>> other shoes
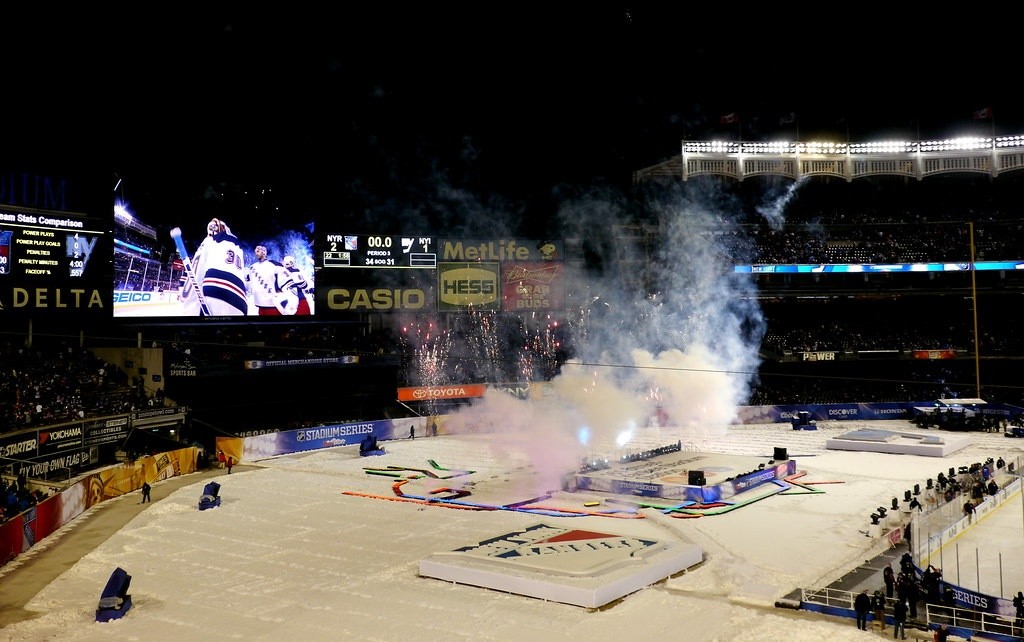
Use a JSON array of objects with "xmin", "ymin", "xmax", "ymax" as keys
[
  {"xmin": 862, "ymin": 628, "xmax": 867, "ymax": 631},
  {"xmin": 902, "ymin": 637, "xmax": 906, "ymax": 640}
]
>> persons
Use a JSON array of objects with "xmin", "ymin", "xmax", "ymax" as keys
[
  {"xmin": 227, "ymin": 457, "xmax": 232, "ymax": 474},
  {"xmin": 432, "ymin": 422, "xmax": 438, "ymax": 437},
  {"xmin": 904, "ymin": 524, "xmax": 911, "ymax": 552},
  {"xmin": 141, "ymin": 482, "xmax": 151, "ymax": 503},
  {"xmin": 913, "ymin": 405, "xmax": 1007, "ymax": 432},
  {"xmin": 715, "ymin": 209, "xmax": 1024, "ymax": 404},
  {"xmin": 113, "ymin": 207, "xmax": 312, "ymax": 315},
  {"xmin": 0, "ymin": 470, "xmax": 48, "ymax": 525},
  {"xmin": 408, "ymin": 425, "xmax": 414, "ymax": 439},
  {"xmin": 854, "ymin": 553, "xmax": 1024, "ymax": 642},
  {"xmin": 908, "ymin": 456, "xmax": 1004, "ymax": 526},
  {"xmin": 197, "ymin": 450, "xmax": 214, "ymax": 469},
  {"xmin": 219, "ymin": 450, "xmax": 226, "ymax": 469},
  {"xmin": 0, "ymin": 343, "xmax": 163, "ymax": 432}
]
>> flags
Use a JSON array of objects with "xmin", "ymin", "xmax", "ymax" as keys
[
  {"xmin": 685, "ymin": 115, "xmax": 706, "ymax": 128},
  {"xmin": 720, "ymin": 113, "xmax": 738, "ymax": 124},
  {"xmin": 974, "ymin": 109, "xmax": 992, "ymax": 118},
  {"xmin": 779, "ymin": 113, "xmax": 795, "ymax": 125}
]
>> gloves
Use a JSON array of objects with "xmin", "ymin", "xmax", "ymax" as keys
[{"xmin": 284, "ymin": 291, "xmax": 299, "ymax": 315}]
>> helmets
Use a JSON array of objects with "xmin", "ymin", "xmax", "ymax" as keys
[
  {"xmin": 283, "ymin": 256, "xmax": 295, "ymax": 268},
  {"xmin": 255, "ymin": 246, "xmax": 266, "ymax": 257},
  {"xmin": 206, "ymin": 218, "xmax": 226, "ymax": 236}
]
